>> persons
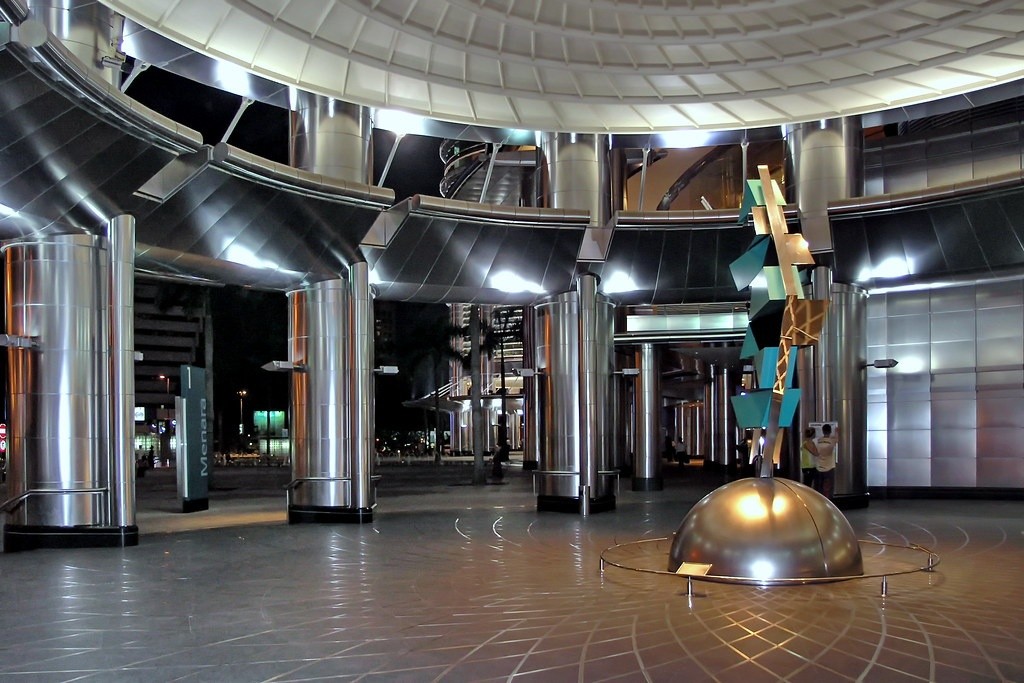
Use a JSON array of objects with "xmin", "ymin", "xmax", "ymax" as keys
[
  {"xmin": 137, "ymin": 445, "xmax": 143, "ymax": 461},
  {"xmin": 491, "ymin": 444, "xmax": 503, "ymax": 482},
  {"xmin": 816, "ymin": 424, "xmax": 839, "ymax": 501},
  {"xmin": 675, "ymin": 436, "xmax": 685, "ymax": 468},
  {"xmin": 148, "ymin": 445, "xmax": 154, "ymax": 469},
  {"xmin": 666, "ymin": 431, "xmax": 673, "ymax": 461},
  {"xmin": 137, "ymin": 456, "xmax": 147, "ymax": 478},
  {"xmin": 801, "ymin": 427, "xmax": 818, "ymax": 488}
]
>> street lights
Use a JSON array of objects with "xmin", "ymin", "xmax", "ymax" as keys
[
  {"xmin": 161, "ymin": 375, "xmax": 169, "ymax": 394},
  {"xmin": 237, "ymin": 391, "xmax": 247, "ymax": 424}
]
